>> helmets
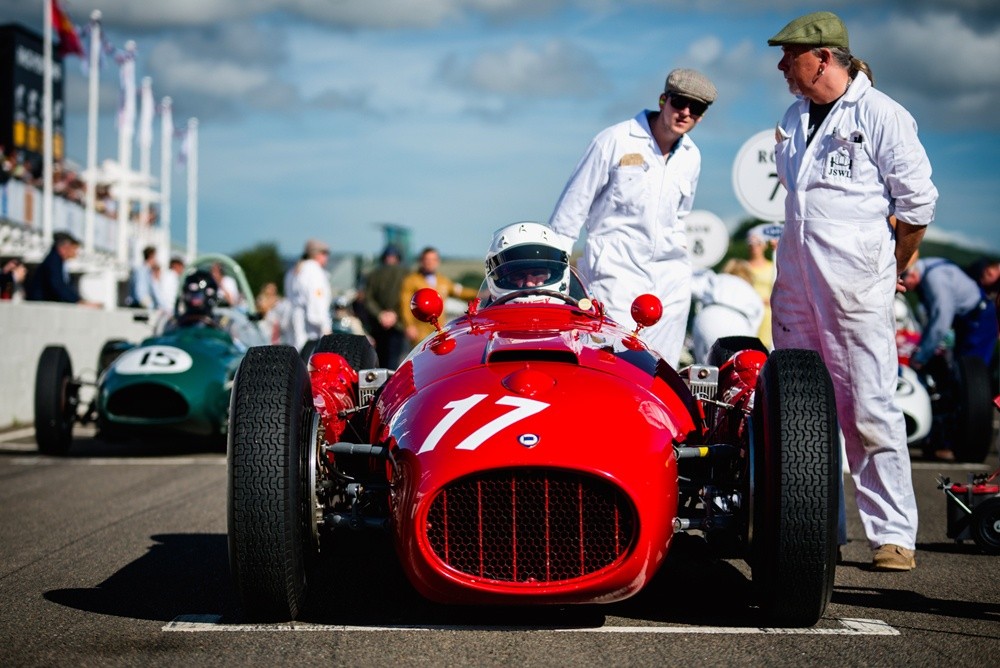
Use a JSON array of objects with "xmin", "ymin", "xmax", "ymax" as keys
[
  {"xmin": 183, "ymin": 272, "xmax": 216, "ymax": 313},
  {"xmin": 484, "ymin": 221, "xmax": 572, "ymax": 306}
]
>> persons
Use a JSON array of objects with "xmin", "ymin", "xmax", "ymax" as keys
[
  {"xmin": 292, "ymin": 240, "xmax": 332, "ymax": 353},
  {"xmin": 28, "ymin": 232, "xmax": 103, "ymax": 307},
  {"xmin": 897, "ymin": 257, "xmax": 1000, "ymax": 376},
  {"xmin": 554, "ymin": 68, "xmax": 716, "ymax": 366},
  {"xmin": 11, "ymin": 265, "xmax": 27, "ymax": 301},
  {"xmin": 691, "ymin": 226, "xmax": 781, "ymax": 367},
  {"xmin": 364, "ymin": 246, "xmax": 410, "ymax": 369},
  {"xmin": 399, "ymin": 247, "xmax": 490, "ymax": 348},
  {"xmin": 131, "ymin": 247, "xmax": 292, "ymax": 345},
  {"xmin": 768, "ymin": 12, "xmax": 938, "ymax": 571},
  {"xmin": 484, "ymin": 222, "xmax": 573, "ymax": 305},
  {"xmin": 332, "ymin": 298, "xmax": 375, "ymax": 347}
]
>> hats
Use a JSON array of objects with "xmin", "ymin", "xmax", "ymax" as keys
[
  {"xmin": 666, "ymin": 69, "xmax": 715, "ymax": 106},
  {"xmin": 307, "ymin": 241, "xmax": 332, "ymax": 255},
  {"xmin": 767, "ymin": 12, "xmax": 849, "ymax": 49}
]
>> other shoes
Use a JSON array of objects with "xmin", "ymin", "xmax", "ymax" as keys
[{"xmin": 873, "ymin": 544, "xmax": 917, "ymax": 571}]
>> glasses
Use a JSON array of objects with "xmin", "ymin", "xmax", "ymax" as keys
[{"xmin": 668, "ymin": 94, "xmax": 708, "ymax": 117}]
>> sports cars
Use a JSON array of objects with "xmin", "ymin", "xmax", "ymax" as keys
[
  {"xmin": 228, "ymin": 255, "xmax": 839, "ymax": 631},
  {"xmin": 32, "ymin": 293, "xmax": 318, "ymax": 451}
]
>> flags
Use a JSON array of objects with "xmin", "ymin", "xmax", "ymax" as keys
[
  {"xmin": 52, "ymin": 0, "xmax": 83, "ymax": 55},
  {"xmin": 98, "ymin": 24, "xmax": 188, "ymax": 161}
]
